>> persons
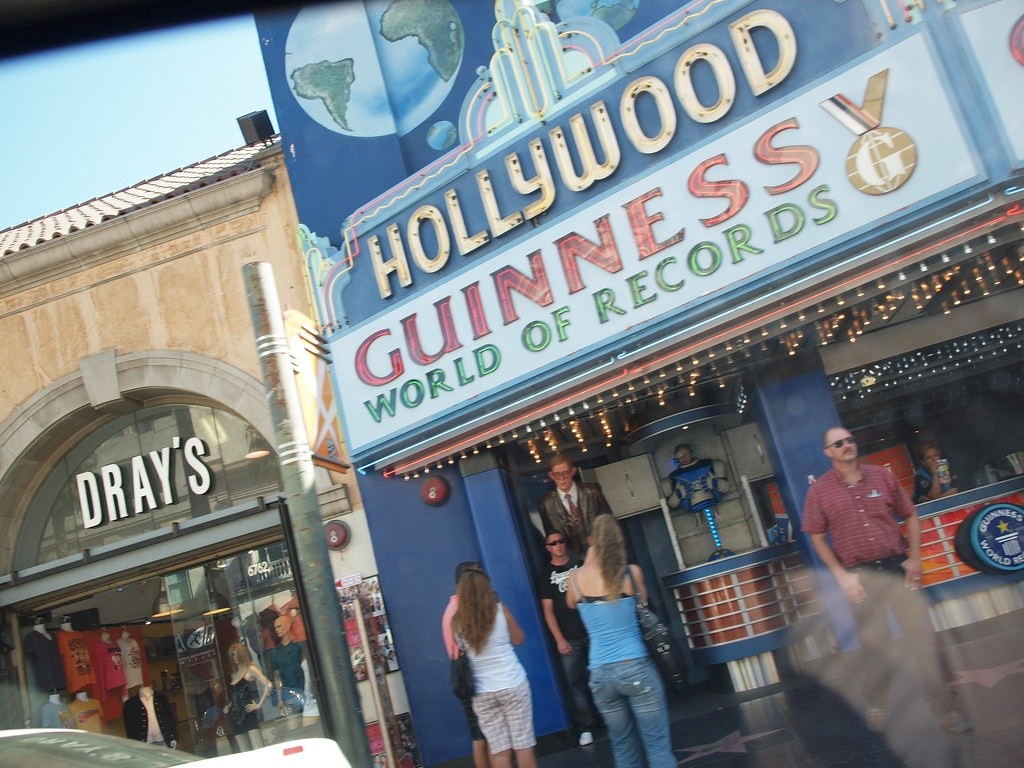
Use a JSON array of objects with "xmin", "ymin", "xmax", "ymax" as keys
[
  {"xmin": 270, "ymin": 617, "xmax": 311, "ymax": 715},
  {"xmin": 912, "ymin": 441, "xmax": 958, "ymax": 503},
  {"xmin": 565, "ymin": 514, "xmax": 690, "ymax": 768},
  {"xmin": 666, "ymin": 443, "xmax": 736, "ymax": 562},
  {"xmin": 222, "ymin": 643, "xmax": 273, "ymax": 753},
  {"xmin": 538, "ymin": 454, "xmax": 614, "ymax": 560},
  {"xmin": 801, "ymin": 426, "xmax": 976, "ymax": 734},
  {"xmin": 441, "ymin": 561, "xmax": 539, "ymax": 768},
  {"xmin": 538, "ymin": 530, "xmax": 597, "ymax": 747},
  {"xmin": 124, "ymin": 686, "xmax": 178, "ymax": 751}
]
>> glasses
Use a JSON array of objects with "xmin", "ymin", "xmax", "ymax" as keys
[
  {"xmin": 545, "ymin": 539, "xmax": 565, "ymax": 546},
  {"xmin": 825, "ymin": 434, "xmax": 855, "ymax": 449},
  {"xmin": 552, "ymin": 468, "xmax": 571, "ymax": 479}
]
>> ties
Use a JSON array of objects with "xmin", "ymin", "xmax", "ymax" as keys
[{"xmin": 565, "ymin": 494, "xmax": 576, "ymax": 515}]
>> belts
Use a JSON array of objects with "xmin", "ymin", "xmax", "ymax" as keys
[{"xmin": 848, "ymin": 554, "xmax": 905, "ymax": 573}]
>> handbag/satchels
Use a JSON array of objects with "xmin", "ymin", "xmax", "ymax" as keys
[
  {"xmin": 627, "ymin": 566, "xmax": 682, "ymax": 694},
  {"xmin": 451, "ymin": 649, "xmax": 473, "ymax": 698}
]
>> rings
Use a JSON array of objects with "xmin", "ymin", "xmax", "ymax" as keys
[{"xmin": 913, "ymin": 574, "xmax": 923, "ymax": 581}]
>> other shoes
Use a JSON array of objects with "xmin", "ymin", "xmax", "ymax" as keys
[
  {"xmin": 862, "ymin": 703, "xmax": 888, "ymax": 734},
  {"xmin": 578, "ymin": 732, "xmax": 593, "ymax": 745},
  {"xmin": 940, "ymin": 707, "xmax": 974, "ymax": 733}
]
